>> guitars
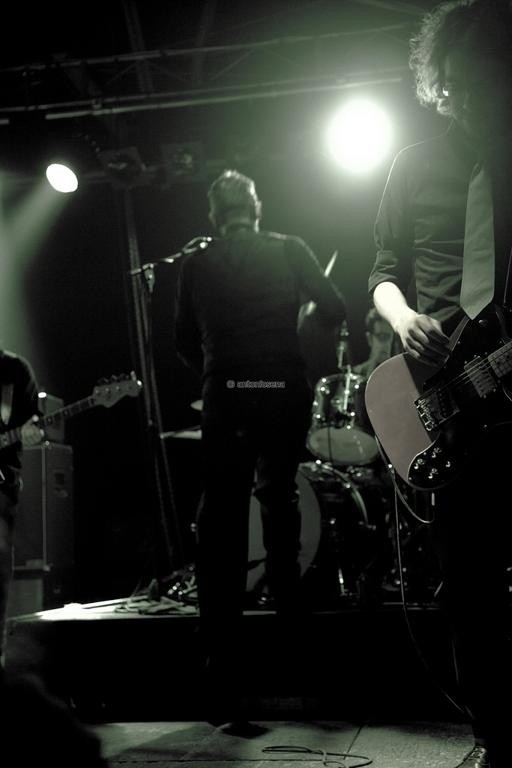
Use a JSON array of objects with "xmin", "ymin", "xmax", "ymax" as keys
[{"xmin": 364, "ymin": 309, "xmax": 511, "ymax": 491}]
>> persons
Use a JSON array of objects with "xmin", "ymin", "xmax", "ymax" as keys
[
  {"xmin": 171, "ymin": 168, "xmax": 347, "ymax": 720},
  {"xmin": 351, "ymin": 311, "xmax": 398, "ymax": 375},
  {"xmin": 1, "ymin": 340, "xmax": 46, "ymax": 644},
  {"xmin": 366, "ymin": 0, "xmax": 512, "ymax": 767}
]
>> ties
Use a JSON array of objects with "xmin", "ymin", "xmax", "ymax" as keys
[{"xmin": 459, "ymin": 140, "xmax": 507, "ymax": 321}]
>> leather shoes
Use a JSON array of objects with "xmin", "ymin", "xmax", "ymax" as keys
[{"xmin": 455, "ymin": 744, "xmax": 490, "ymax": 768}]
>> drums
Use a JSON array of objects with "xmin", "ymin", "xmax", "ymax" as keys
[
  {"xmin": 194, "ymin": 461, "xmax": 367, "ymax": 596},
  {"xmin": 309, "ymin": 373, "xmax": 378, "ymax": 466}
]
